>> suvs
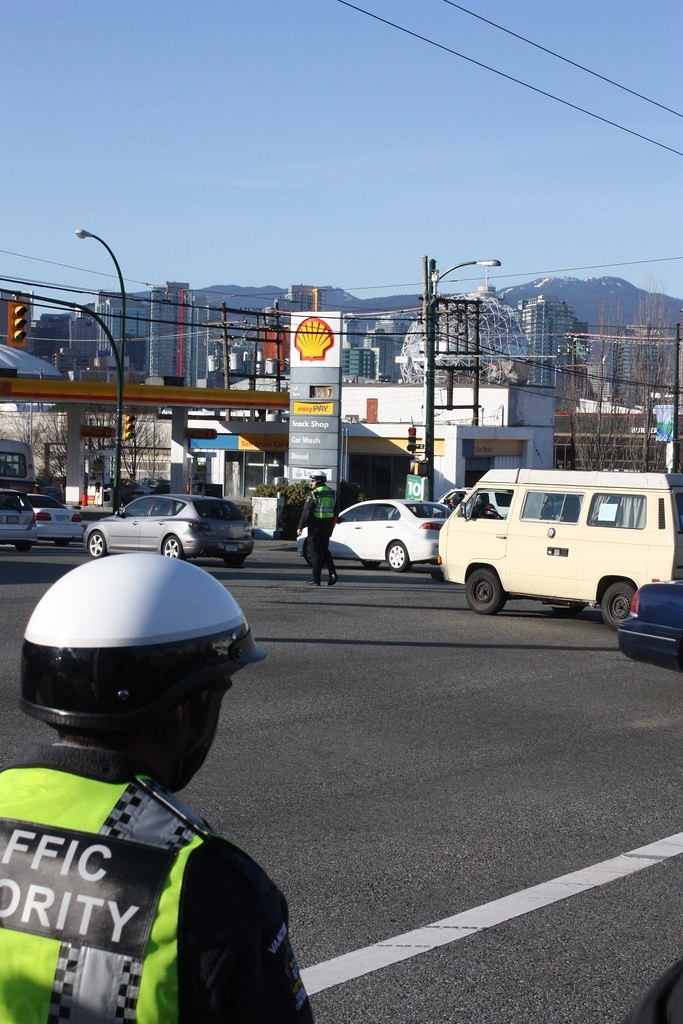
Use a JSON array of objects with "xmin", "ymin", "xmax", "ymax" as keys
[{"xmin": 432, "ymin": 487, "xmax": 516, "ymax": 519}]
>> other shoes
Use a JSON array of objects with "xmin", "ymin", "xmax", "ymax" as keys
[
  {"xmin": 309, "ymin": 581, "xmax": 321, "ymax": 586},
  {"xmin": 328, "ymin": 573, "xmax": 338, "ymax": 585}
]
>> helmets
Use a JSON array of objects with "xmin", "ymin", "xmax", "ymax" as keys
[
  {"xmin": 20, "ymin": 552, "xmax": 269, "ymax": 735},
  {"xmin": 309, "ymin": 470, "xmax": 326, "ymax": 482}
]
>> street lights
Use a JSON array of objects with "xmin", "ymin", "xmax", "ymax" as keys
[
  {"xmin": 75, "ymin": 228, "xmax": 124, "ymax": 515},
  {"xmin": 419, "ymin": 253, "xmax": 502, "ymax": 503}
]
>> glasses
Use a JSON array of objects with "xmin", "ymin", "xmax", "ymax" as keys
[{"xmin": 311, "ymin": 479, "xmax": 317, "ymax": 482}]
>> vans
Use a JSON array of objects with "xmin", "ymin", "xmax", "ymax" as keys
[{"xmin": 435, "ymin": 469, "xmax": 683, "ymax": 631}]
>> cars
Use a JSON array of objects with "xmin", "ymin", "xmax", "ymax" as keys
[
  {"xmin": 105, "ymin": 476, "xmax": 155, "ymax": 507},
  {"xmin": 0, "ymin": 489, "xmax": 40, "ymax": 551},
  {"xmin": 42, "ymin": 486, "xmax": 65, "ymax": 502},
  {"xmin": 297, "ymin": 498, "xmax": 452, "ymax": 573},
  {"xmin": 617, "ymin": 579, "xmax": 683, "ymax": 672},
  {"xmin": 29, "ymin": 493, "xmax": 85, "ymax": 548},
  {"xmin": 83, "ymin": 493, "xmax": 255, "ymax": 568}
]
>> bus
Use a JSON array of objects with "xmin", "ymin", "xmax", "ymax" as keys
[{"xmin": 0, "ymin": 438, "xmax": 37, "ymax": 493}]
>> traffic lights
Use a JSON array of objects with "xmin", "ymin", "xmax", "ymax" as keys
[
  {"xmin": 406, "ymin": 428, "xmax": 416, "ymax": 452},
  {"xmin": 7, "ymin": 300, "xmax": 28, "ymax": 347},
  {"xmin": 125, "ymin": 416, "xmax": 142, "ymax": 444}
]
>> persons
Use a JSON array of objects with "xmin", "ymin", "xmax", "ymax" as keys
[
  {"xmin": 296, "ymin": 470, "xmax": 340, "ymax": 586},
  {"xmin": 0, "ymin": 550, "xmax": 315, "ymax": 1024}
]
